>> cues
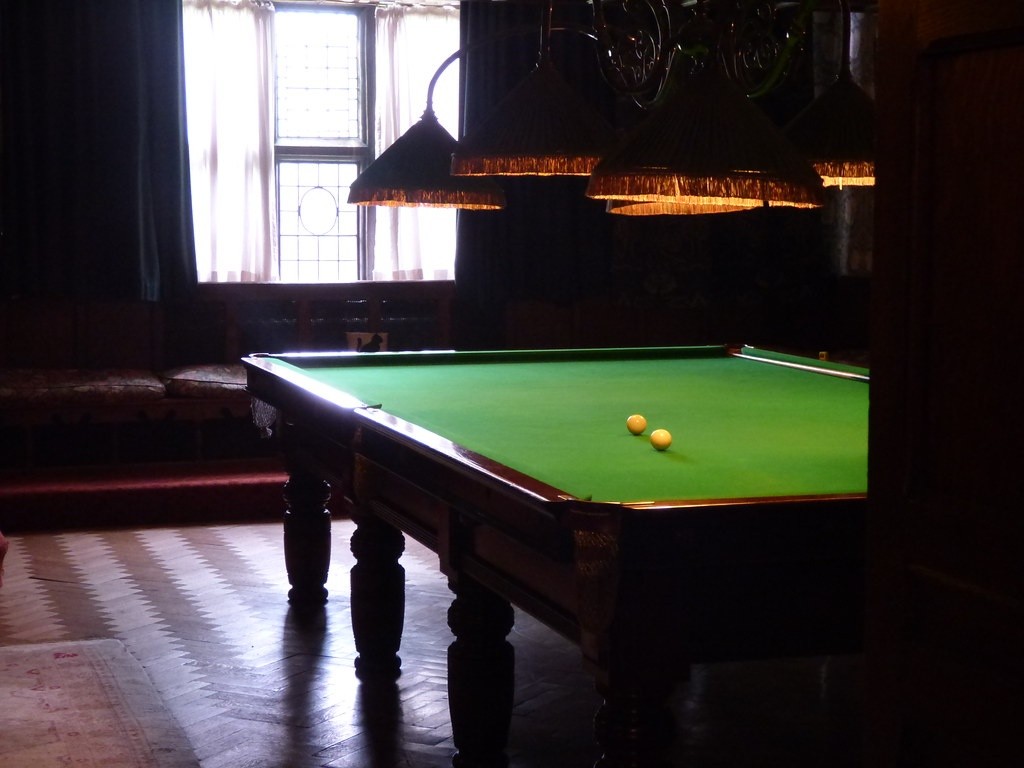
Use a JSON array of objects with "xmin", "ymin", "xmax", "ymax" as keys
[{"xmin": 732, "ymin": 352, "xmax": 870, "ymax": 383}]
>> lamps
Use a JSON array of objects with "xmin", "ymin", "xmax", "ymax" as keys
[{"xmin": 347, "ymin": 0, "xmax": 878, "ymax": 218}]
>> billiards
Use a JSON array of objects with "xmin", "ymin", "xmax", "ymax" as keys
[
  {"xmin": 626, "ymin": 414, "xmax": 647, "ymax": 435},
  {"xmin": 650, "ymin": 429, "xmax": 672, "ymax": 451}
]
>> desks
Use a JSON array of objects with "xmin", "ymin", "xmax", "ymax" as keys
[{"xmin": 240, "ymin": 339, "xmax": 871, "ymax": 768}]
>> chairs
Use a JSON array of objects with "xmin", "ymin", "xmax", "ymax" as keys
[
  {"xmin": 366, "ymin": 281, "xmax": 457, "ymax": 350},
  {"xmin": 295, "ymin": 293, "xmax": 377, "ymax": 348},
  {"xmin": 69, "ymin": 290, "xmax": 166, "ymax": 464},
  {"xmin": 241, "ymin": 283, "xmax": 295, "ymax": 425},
  {"xmin": 0, "ymin": 292, "xmax": 69, "ymax": 467},
  {"xmin": 152, "ymin": 282, "xmax": 251, "ymax": 462}
]
